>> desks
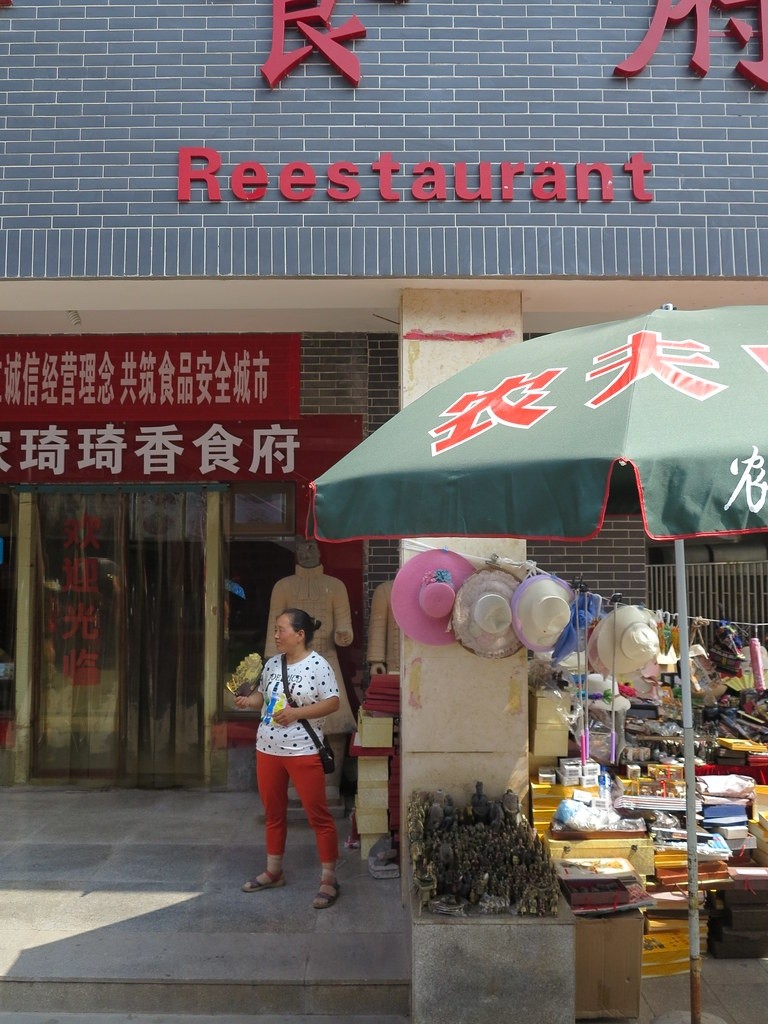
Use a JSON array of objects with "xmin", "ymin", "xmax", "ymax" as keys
[{"xmin": 619, "ymin": 764, "xmax": 768, "ymax": 786}]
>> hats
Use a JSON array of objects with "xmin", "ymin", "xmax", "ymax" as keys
[
  {"xmin": 579, "ymin": 672, "xmax": 631, "ymax": 712},
  {"xmin": 511, "ymin": 575, "xmax": 575, "ymax": 652},
  {"xmin": 392, "ymin": 549, "xmax": 479, "ymax": 646},
  {"xmin": 451, "ymin": 566, "xmax": 523, "ymax": 658},
  {"xmin": 586, "ymin": 605, "xmax": 661, "ymax": 681}
]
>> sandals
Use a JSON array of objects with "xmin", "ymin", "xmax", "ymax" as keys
[
  {"xmin": 242, "ymin": 867, "xmax": 285, "ymax": 893},
  {"xmin": 312, "ymin": 877, "xmax": 340, "ymax": 908}
]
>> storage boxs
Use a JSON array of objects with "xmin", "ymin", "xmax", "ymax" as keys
[
  {"xmin": 560, "ymin": 878, "xmax": 630, "ymax": 906},
  {"xmin": 715, "ymin": 921, "xmax": 768, "ymax": 944},
  {"xmin": 538, "ymin": 767, "xmax": 557, "ymax": 785},
  {"xmin": 555, "ymin": 768, "xmax": 580, "ymax": 787},
  {"xmin": 576, "ymin": 908, "xmax": 646, "ymax": 1020},
  {"xmin": 541, "ymin": 830, "xmax": 656, "ymax": 877},
  {"xmin": 626, "ymin": 765, "xmax": 641, "ymax": 780},
  {"xmin": 725, "ymin": 905, "xmax": 768, "ymax": 933},
  {"xmin": 559, "ymin": 759, "xmax": 583, "ymax": 778},
  {"xmin": 579, "ymin": 775, "xmax": 598, "ymax": 788},
  {"xmin": 529, "ymin": 721, "xmax": 569, "ymax": 757},
  {"xmin": 709, "ymin": 936, "xmax": 768, "ymax": 960},
  {"xmin": 527, "ymin": 685, "xmax": 572, "ymax": 725},
  {"xmin": 357, "ymin": 706, "xmax": 393, "ymax": 748},
  {"xmin": 353, "ymin": 795, "xmax": 388, "ymax": 835},
  {"xmin": 718, "ymin": 889, "xmax": 768, "ymax": 906},
  {"xmin": 357, "ymin": 756, "xmax": 389, "ymax": 782},
  {"xmin": 357, "ymin": 782, "xmax": 388, "ymax": 808},
  {"xmin": 360, "ymin": 834, "xmax": 381, "ymax": 861},
  {"xmin": 578, "ymin": 758, "xmax": 601, "ymax": 776}
]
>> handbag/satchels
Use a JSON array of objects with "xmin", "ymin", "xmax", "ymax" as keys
[{"xmin": 318, "ymin": 735, "xmax": 336, "ymax": 774}]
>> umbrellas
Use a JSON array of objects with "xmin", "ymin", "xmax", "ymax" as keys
[{"xmin": 303, "ymin": 304, "xmax": 768, "ymax": 1024}]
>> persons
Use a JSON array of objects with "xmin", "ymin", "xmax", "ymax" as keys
[
  {"xmin": 231, "ymin": 607, "xmax": 342, "ymax": 909},
  {"xmin": 263, "ymin": 531, "xmax": 358, "ymax": 804}
]
